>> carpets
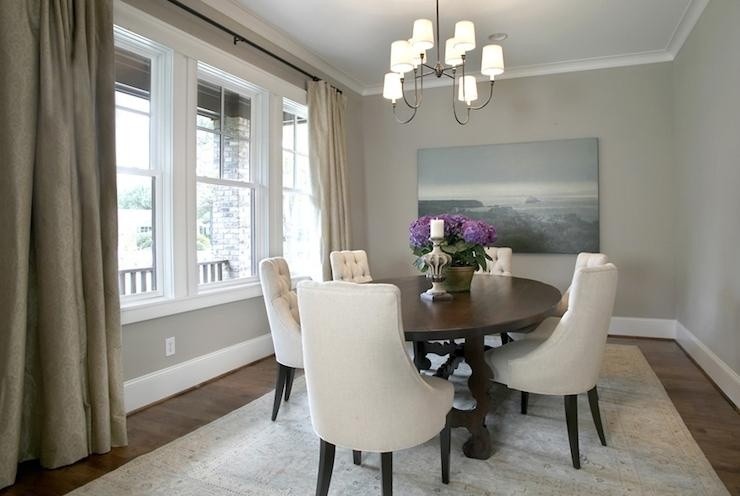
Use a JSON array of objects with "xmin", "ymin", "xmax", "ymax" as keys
[{"xmin": 60, "ymin": 341, "xmax": 731, "ymax": 495}]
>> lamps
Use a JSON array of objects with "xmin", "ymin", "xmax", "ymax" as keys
[{"xmin": 380, "ymin": 1, "xmax": 512, "ymax": 131}]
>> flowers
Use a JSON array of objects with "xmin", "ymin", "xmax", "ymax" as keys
[{"xmin": 408, "ymin": 211, "xmax": 498, "ymax": 275}]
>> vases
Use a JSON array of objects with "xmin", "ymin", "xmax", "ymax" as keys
[{"xmin": 439, "ymin": 265, "xmax": 476, "ymax": 292}]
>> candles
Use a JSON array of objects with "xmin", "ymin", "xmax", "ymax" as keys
[{"xmin": 430, "ymin": 216, "xmax": 445, "ymax": 239}]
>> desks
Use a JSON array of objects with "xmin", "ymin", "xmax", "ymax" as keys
[{"xmin": 356, "ymin": 273, "xmax": 563, "ymax": 463}]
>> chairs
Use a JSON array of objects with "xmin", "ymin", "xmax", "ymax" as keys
[
  {"xmin": 256, "ymin": 255, "xmax": 307, "ymax": 424},
  {"xmin": 471, "ymin": 247, "xmax": 516, "ymax": 344},
  {"xmin": 329, "ymin": 248, "xmax": 373, "ymax": 287},
  {"xmin": 484, "ymin": 262, "xmax": 619, "ymax": 472},
  {"xmin": 501, "ymin": 250, "xmax": 608, "ymax": 352},
  {"xmin": 295, "ymin": 279, "xmax": 455, "ymax": 496}
]
